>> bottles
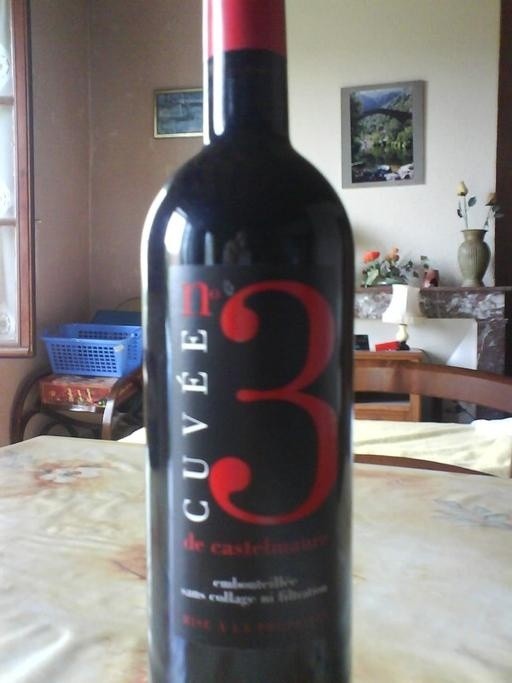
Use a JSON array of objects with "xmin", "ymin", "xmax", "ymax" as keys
[{"xmin": 141, "ymin": 2, "xmax": 353, "ymax": 683}]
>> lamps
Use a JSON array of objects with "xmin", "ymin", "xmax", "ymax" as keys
[{"xmin": 381, "ymin": 283, "xmax": 428, "ymax": 351}]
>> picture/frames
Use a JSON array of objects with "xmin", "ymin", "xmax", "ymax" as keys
[
  {"xmin": 151, "ymin": 88, "xmax": 204, "ymax": 140},
  {"xmin": 340, "ymin": 80, "xmax": 425, "ymax": 190}
]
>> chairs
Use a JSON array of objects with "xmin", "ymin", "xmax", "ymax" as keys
[{"xmin": 9, "ymin": 297, "xmax": 143, "ymax": 444}]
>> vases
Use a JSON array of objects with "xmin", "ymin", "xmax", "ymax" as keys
[{"xmin": 458, "ymin": 229, "xmax": 491, "ymax": 287}]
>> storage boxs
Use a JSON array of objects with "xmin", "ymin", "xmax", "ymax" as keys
[{"xmin": 37, "ymin": 366, "xmax": 143, "ymax": 408}]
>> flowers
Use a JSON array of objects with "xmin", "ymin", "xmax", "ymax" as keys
[
  {"xmin": 456, "ymin": 180, "xmax": 504, "ymax": 229},
  {"xmin": 360, "ymin": 244, "xmax": 439, "ymax": 287}
]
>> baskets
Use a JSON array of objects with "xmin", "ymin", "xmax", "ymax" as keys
[
  {"xmin": 38, "ymin": 322, "xmax": 143, "ymax": 378},
  {"xmin": 91, "ymin": 308, "xmax": 142, "ymax": 326}
]
